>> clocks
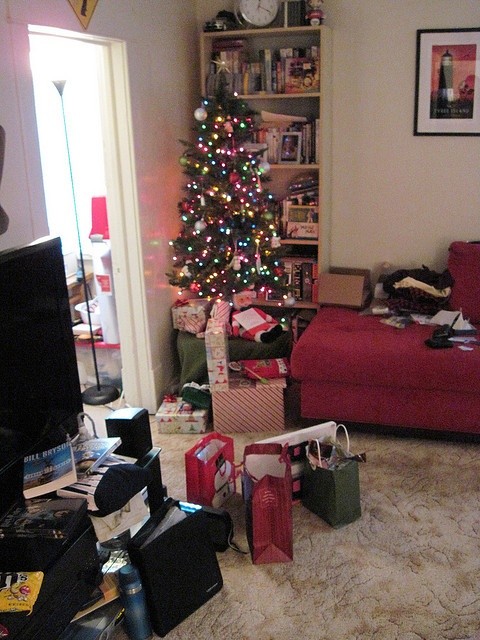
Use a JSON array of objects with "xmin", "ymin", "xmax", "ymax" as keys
[{"xmin": 234, "ymin": 0, "xmax": 281, "ymax": 29}]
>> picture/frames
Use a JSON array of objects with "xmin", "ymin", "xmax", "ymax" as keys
[{"xmin": 413, "ymin": 27, "xmax": 480, "ymax": 137}]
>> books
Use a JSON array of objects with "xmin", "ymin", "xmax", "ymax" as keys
[
  {"xmin": 253, "ymin": 109, "xmax": 320, "ymax": 165},
  {"xmin": 1, "ymin": 498, "xmax": 88, "ymax": 541},
  {"xmin": 266, "ymin": 253, "xmax": 317, "ymax": 302},
  {"xmin": 22, "ymin": 442, "xmax": 78, "ymax": 500},
  {"xmin": 275, "ymin": 200, "xmax": 318, "ymax": 239},
  {"xmin": 206, "ymin": 39, "xmax": 318, "ymax": 94},
  {"xmin": 71, "ymin": 572, "xmax": 120, "ymax": 623},
  {"xmin": 67, "ymin": 436, "xmax": 123, "ymax": 478},
  {"xmin": 57, "ymin": 453, "xmax": 138, "ymax": 511}
]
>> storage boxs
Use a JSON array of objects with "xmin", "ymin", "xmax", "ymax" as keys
[
  {"xmin": 212, "ymin": 372, "xmax": 287, "ymax": 436},
  {"xmin": 154, "ymin": 397, "xmax": 208, "ymax": 433}
]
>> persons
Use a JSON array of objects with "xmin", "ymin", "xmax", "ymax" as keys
[{"xmin": 232, "ymin": 291, "xmax": 282, "ymax": 344}]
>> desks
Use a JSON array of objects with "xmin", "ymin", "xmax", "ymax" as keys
[
  {"xmin": 175, "ymin": 329, "xmax": 289, "ymax": 384},
  {"xmin": 0, "ymin": 446, "xmax": 164, "ymax": 640}
]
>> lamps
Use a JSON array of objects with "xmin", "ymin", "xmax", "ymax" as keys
[{"xmin": 53, "ymin": 79, "xmax": 120, "ymax": 405}]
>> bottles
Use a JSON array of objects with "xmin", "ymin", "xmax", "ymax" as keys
[{"xmin": 118, "ymin": 566, "xmax": 152, "ymax": 640}]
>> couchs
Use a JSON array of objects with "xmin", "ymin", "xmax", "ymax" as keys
[{"xmin": 291, "ymin": 298, "xmax": 480, "ymax": 434}]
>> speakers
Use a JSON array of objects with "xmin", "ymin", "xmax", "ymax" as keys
[
  {"xmin": 105, "ymin": 408, "xmax": 153, "ymax": 460},
  {"xmin": 136, "ymin": 450, "xmax": 164, "ymax": 514}
]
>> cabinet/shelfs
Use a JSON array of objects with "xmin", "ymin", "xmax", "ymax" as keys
[{"xmin": 200, "ymin": 26, "xmax": 333, "ymax": 310}]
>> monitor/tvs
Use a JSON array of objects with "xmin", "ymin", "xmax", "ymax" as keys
[{"xmin": 0, "ymin": 235, "xmax": 84, "ymax": 513}]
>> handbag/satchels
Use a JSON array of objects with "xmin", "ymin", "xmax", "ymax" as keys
[
  {"xmin": 301, "ymin": 424, "xmax": 362, "ymax": 530},
  {"xmin": 241, "ymin": 421, "xmax": 336, "ymax": 505},
  {"xmin": 192, "ymin": 508, "xmax": 251, "ymax": 559},
  {"xmin": 244, "ymin": 444, "xmax": 292, "ymax": 565},
  {"xmin": 386, "ymin": 266, "xmax": 452, "ymax": 316},
  {"xmin": 185, "ymin": 432, "xmax": 237, "ymax": 509}
]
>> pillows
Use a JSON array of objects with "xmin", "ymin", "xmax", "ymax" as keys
[{"xmin": 447, "ymin": 241, "xmax": 480, "ymax": 325}]
